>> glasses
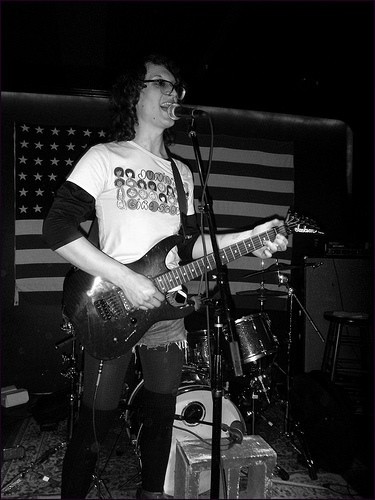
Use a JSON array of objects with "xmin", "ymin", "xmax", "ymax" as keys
[{"xmin": 142, "ymin": 79, "xmax": 186, "ymax": 100}]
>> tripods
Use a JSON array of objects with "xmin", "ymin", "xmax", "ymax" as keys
[
  {"xmin": 1, "ymin": 336, "xmax": 115, "ymax": 500},
  {"xmin": 271, "ymin": 268, "xmax": 317, "ymax": 481}
]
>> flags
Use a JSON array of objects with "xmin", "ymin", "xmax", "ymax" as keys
[{"xmin": 13, "ymin": 120, "xmax": 295, "ymax": 310}]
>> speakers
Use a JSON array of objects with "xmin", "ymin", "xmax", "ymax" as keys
[{"xmin": 293, "ymin": 256, "xmax": 375, "ymax": 377}]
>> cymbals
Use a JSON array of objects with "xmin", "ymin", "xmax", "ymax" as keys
[
  {"xmin": 236, "ymin": 282, "xmax": 290, "ymax": 299},
  {"xmin": 242, "ymin": 259, "xmax": 315, "ymax": 279}
]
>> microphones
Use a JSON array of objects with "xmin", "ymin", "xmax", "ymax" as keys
[
  {"xmin": 167, "ymin": 103, "xmax": 207, "ymax": 121},
  {"xmin": 230, "ymin": 420, "xmax": 246, "ymax": 444}
]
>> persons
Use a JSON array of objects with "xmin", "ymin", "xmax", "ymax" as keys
[{"xmin": 43, "ymin": 50, "xmax": 290, "ymax": 500}]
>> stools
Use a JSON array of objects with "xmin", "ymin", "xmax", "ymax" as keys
[
  {"xmin": 325, "ymin": 311, "xmax": 374, "ymax": 387},
  {"xmin": 174, "ymin": 435, "xmax": 277, "ymax": 500}
]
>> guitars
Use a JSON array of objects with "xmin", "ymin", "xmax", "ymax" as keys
[{"xmin": 61, "ymin": 208, "xmax": 324, "ymax": 361}]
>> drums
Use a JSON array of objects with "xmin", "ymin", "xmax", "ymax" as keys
[
  {"xmin": 120, "ymin": 365, "xmax": 229, "ymax": 445},
  {"xmin": 234, "ymin": 309, "xmax": 281, "ymax": 363},
  {"xmin": 130, "ymin": 364, "xmax": 248, "ymax": 497},
  {"xmin": 186, "ymin": 329, "xmax": 219, "ymax": 378}
]
